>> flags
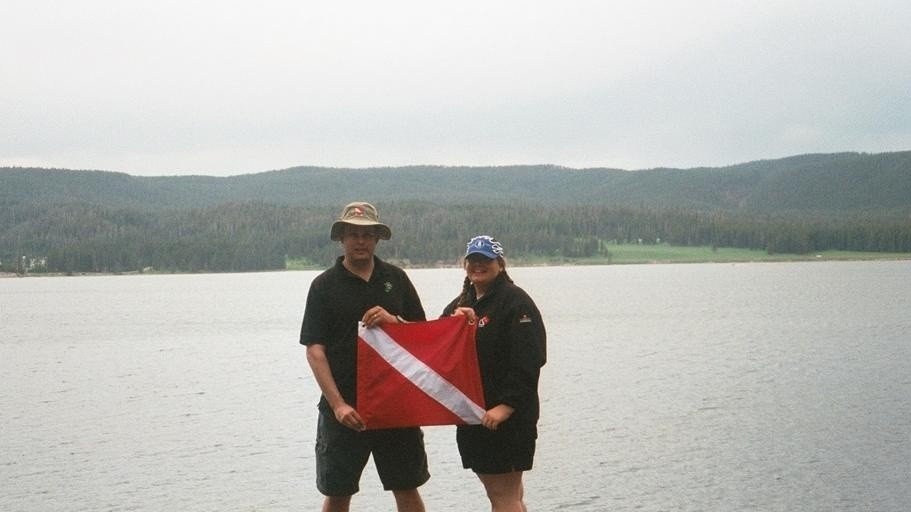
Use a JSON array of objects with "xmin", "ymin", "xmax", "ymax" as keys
[{"xmin": 356, "ymin": 315, "xmax": 487, "ymax": 432}]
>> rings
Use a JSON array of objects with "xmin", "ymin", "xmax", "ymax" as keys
[{"xmin": 374, "ymin": 313, "xmax": 377, "ymax": 317}]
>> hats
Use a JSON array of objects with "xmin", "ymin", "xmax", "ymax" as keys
[
  {"xmin": 331, "ymin": 201, "xmax": 391, "ymax": 241},
  {"xmin": 463, "ymin": 235, "xmax": 504, "ymax": 261}
]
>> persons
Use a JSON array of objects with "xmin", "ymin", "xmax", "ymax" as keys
[
  {"xmin": 300, "ymin": 200, "xmax": 432, "ymax": 511},
  {"xmin": 437, "ymin": 234, "xmax": 548, "ymax": 511}
]
